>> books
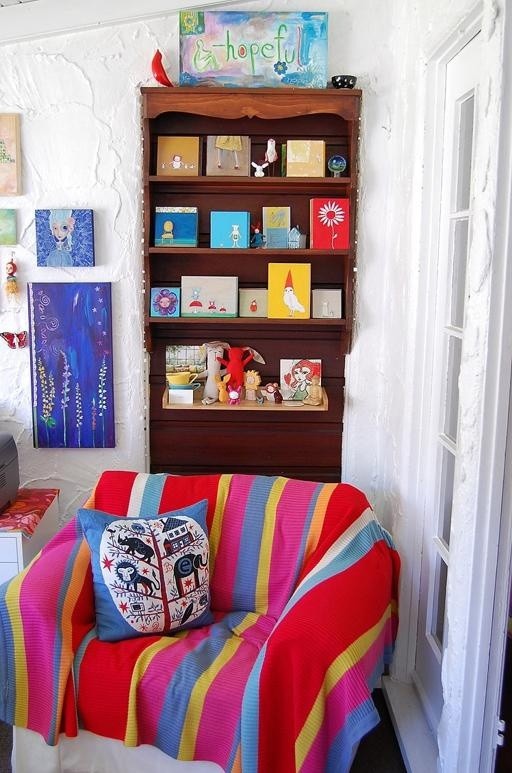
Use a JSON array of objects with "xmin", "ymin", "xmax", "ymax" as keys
[
  {"xmin": 286, "ymin": 139, "xmax": 326, "ymax": 177},
  {"xmin": 206, "ymin": 135, "xmax": 251, "ymax": 176},
  {"xmin": 209, "ymin": 212, "xmax": 250, "ymax": 248},
  {"xmin": 179, "ymin": 275, "xmax": 239, "ymax": 318},
  {"xmin": 154, "ymin": 207, "xmax": 198, "ymax": 248},
  {"xmin": 315, "ymin": 289, "xmax": 344, "ymax": 319},
  {"xmin": 239, "ymin": 287, "xmax": 268, "ymax": 318},
  {"xmin": 309, "ymin": 198, "xmax": 349, "ymax": 251},
  {"xmin": 157, "ymin": 135, "xmax": 203, "ymax": 177},
  {"xmin": 269, "ymin": 263, "xmax": 312, "ymax": 320},
  {"xmin": 263, "ymin": 207, "xmax": 290, "ymax": 250}
]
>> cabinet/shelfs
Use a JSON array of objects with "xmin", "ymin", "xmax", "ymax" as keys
[{"xmin": 141, "ymin": 86, "xmax": 363, "ymax": 482}]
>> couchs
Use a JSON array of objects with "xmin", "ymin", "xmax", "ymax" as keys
[{"xmin": 1, "ymin": 469, "xmax": 401, "ymax": 773}]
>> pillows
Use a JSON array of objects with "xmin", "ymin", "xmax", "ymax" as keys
[{"xmin": 77, "ymin": 498, "xmax": 215, "ymax": 641}]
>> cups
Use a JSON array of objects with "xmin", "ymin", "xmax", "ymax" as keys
[
  {"xmin": 166, "ymin": 372, "xmax": 199, "ymax": 383},
  {"xmin": 168, "ymin": 383, "xmax": 201, "ymax": 390}
]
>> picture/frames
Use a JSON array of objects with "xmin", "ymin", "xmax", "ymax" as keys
[
  {"xmin": 178, "ymin": 7, "xmax": 330, "ymax": 87},
  {"xmin": 27, "ymin": 281, "xmax": 116, "ymax": 448},
  {"xmin": 34, "ymin": 208, "xmax": 97, "ymax": 268},
  {"xmin": 0, "ymin": 112, "xmax": 23, "ymax": 197}
]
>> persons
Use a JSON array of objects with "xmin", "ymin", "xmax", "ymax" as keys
[
  {"xmin": 290, "ymin": 361, "xmax": 321, "ymax": 400},
  {"xmin": 215, "ymin": 136, "xmax": 243, "ymax": 169},
  {"xmin": 46, "ymin": 210, "xmax": 76, "ymax": 267}
]
